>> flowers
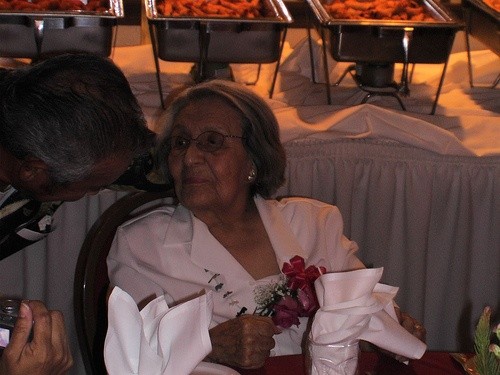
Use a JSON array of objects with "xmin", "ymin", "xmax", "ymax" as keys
[
  {"xmin": 254, "ymin": 254, "xmax": 324, "ymax": 331},
  {"xmin": 473, "ymin": 303, "xmax": 500, "ymax": 375}
]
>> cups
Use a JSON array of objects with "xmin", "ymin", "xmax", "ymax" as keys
[{"xmin": 303, "ymin": 327, "xmax": 360, "ymax": 375}]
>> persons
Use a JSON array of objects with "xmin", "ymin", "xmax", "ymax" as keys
[
  {"xmin": 0, "ymin": 54, "xmax": 173, "ymax": 375},
  {"xmin": 106, "ymin": 79, "xmax": 427, "ymax": 370}
]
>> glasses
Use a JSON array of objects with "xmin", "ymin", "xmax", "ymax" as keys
[{"xmin": 168, "ymin": 125, "xmax": 243, "ymax": 154}]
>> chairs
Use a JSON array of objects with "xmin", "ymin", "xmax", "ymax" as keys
[{"xmin": 73, "ymin": 190, "xmax": 173, "ymax": 375}]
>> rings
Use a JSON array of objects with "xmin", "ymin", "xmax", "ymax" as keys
[{"xmin": 415, "ymin": 324, "xmax": 421, "ymax": 329}]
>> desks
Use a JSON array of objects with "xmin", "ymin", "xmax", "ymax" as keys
[{"xmin": 0, "ymin": 28, "xmax": 500, "ymax": 375}]
